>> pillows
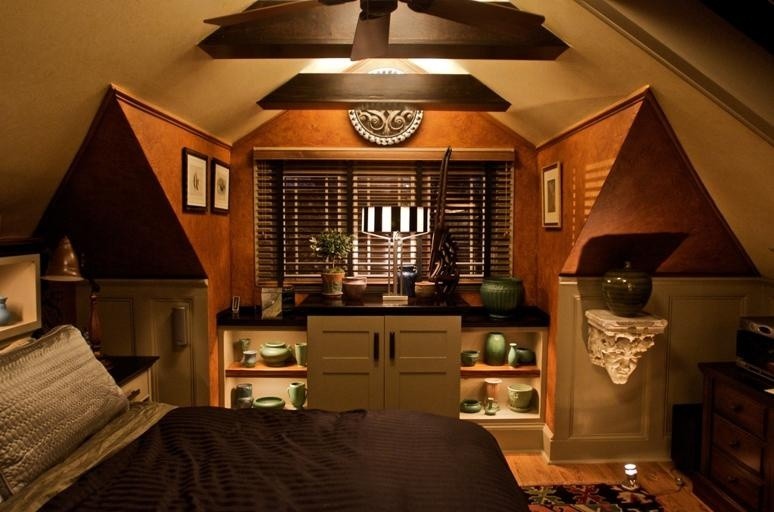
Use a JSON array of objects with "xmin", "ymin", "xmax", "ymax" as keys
[{"xmin": 0, "ymin": 321, "xmax": 127, "ymax": 497}]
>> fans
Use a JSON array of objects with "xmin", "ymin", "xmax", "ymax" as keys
[{"xmin": 203, "ymin": 1, "xmax": 546, "ymax": 61}]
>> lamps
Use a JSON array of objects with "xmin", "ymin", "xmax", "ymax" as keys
[
  {"xmin": 39, "ymin": 235, "xmax": 116, "ymax": 371},
  {"xmin": 360, "ymin": 204, "xmax": 432, "ymax": 300},
  {"xmin": 619, "ymin": 460, "xmax": 642, "ymax": 494}
]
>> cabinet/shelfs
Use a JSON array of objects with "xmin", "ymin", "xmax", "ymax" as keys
[
  {"xmin": 305, "ymin": 313, "xmax": 462, "ymax": 419},
  {"xmin": 460, "ymin": 324, "xmax": 545, "ymax": 452},
  {"xmin": 692, "ymin": 358, "xmax": 772, "ymax": 512},
  {"xmin": 216, "ymin": 319, "xmax": 306, "ymax": 419}
]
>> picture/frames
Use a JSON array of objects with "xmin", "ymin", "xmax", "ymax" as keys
[
  {"xmin": 210, "ymin": 154, "xmax": 232, "ymax": 216},
  {"xmin": 538, "ymin": 157, "xmax": 564, "ymax": 231},
  {"xmin": 183, "ymin": 147, "xmax": 209, "ymax": 212}
]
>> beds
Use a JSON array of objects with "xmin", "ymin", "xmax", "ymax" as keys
[{"xmin": 0, "ymin": 325, "xmax": 533, "ymax": 512}]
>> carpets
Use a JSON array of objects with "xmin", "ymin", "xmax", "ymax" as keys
[{"xmin": 515, "ymin": 480, "xmax": 664, "ymax": 512}]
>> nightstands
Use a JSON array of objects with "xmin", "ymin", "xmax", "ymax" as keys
[{"xmin": 96, "ymin": 349, "xmax": 166, "ymax": 404}]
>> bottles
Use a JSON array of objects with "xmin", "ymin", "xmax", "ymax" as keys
[
  {"xmin": 508, "ymin": 343, "xmax": 518, "ymax": 366},
  {"xmin": 0, "ymin": 296, "xmax": 11, "ymax": 326},
  {"xmin": 461, "ymin": 400, "xmax": 481, "ymax": 413},
  {"xmin": 599, "ymin": 259, "xmax": 652, "ymax": 318},
  {"xmin": 397, "ymin": 266, "xmax": 417, "ymax": 296}
]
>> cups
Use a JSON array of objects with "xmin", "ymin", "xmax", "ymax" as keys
[
  {"xmin": 460, "ymin": 350, "xmax": 479, "ymax": 367},
  {"xmin": 295, "ymin": 343, "xmax": 307, "ymax": 367},
  {"xmin": 478, "ymin": 278, "xmax": 524, "ymax": 319},
  {"xmin": 287, "ymin": 382, "xmax": 307, "ymax": 409},
  {"xmin": 507, "ymin": 384, "xmax": 532, "ymax": 409},
  {"xmin": 243, "ymin": 351, "xmax": 256, "ymax": 366},
  {"xmin": 516, "ymin": 348, "xmax": 532, "ymax": 365},
  {"xmin": 484, "ymin": 379, "xmax": 502, "ymax": 408},
  {"xmin": 239, "ymin": 338, "xmax": 250, "ymax": 364}
]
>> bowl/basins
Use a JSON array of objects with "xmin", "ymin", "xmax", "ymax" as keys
[
  {"xmin": 414, "ymin": 282, "xmax": 435, "ymax": 297},
  {"xmin": 252, "ymin": 396, "xmax": 286, "ymax": 410},
  {"xmin": 342, "ymin": 277, "xmax": 367, "ymax": 299}
]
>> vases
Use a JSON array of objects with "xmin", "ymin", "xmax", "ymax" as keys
[{"xmin": 506, "ymin": 342, "xmax": 519, "ymax": 367}]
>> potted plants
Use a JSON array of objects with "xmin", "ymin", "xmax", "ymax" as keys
[{"xmin": 309, "ymin": 229, "xmax": 353, "ymax": 298}]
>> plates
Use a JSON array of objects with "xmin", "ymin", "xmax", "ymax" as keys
[{"xmin": 506, "ymin": 406, "xmax": 531, "ymax": 413}]
